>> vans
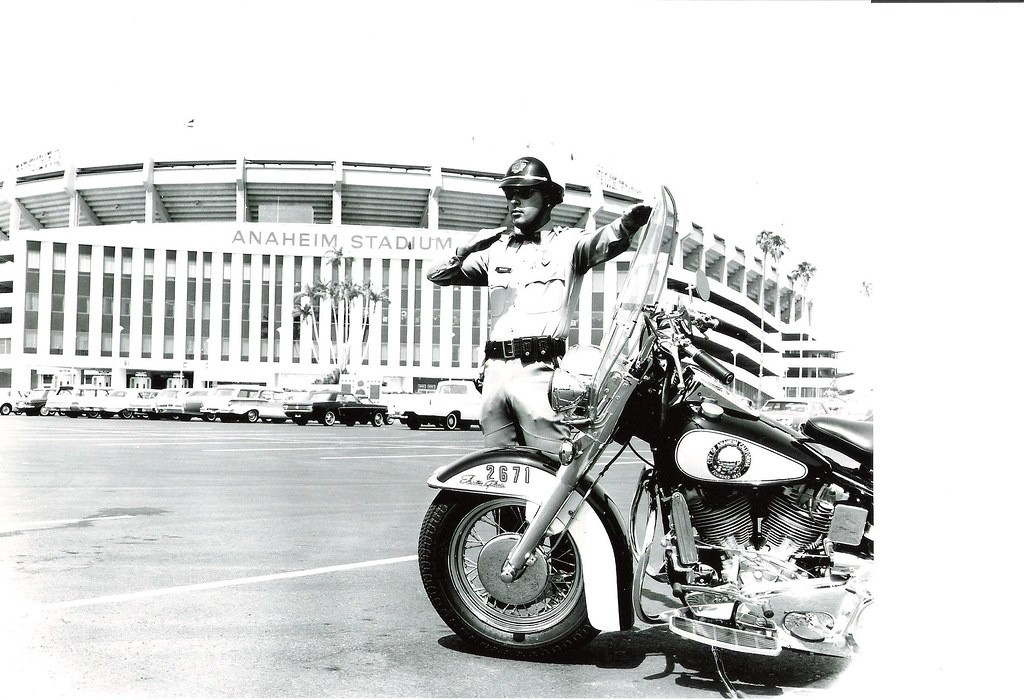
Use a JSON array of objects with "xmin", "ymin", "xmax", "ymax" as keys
[{"xmin": 760, "ymin": 396, "xmax": 851, "ymax": 433}]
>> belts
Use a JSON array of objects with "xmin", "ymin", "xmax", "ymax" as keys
[{"xmin": 485, "ymin": 336, "xmax": 565, "ymax": 362}]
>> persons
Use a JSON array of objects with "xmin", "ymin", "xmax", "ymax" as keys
[{"xmin": 426, "ymin": 156, "xmax": 654, "ymax": 612}]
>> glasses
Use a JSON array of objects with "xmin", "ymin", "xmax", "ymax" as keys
[{"xmin": 505, "ymin": 188, "xmax": 542, "ymax": 199}]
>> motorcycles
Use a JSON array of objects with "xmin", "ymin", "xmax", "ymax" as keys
[{"xmin": 419, "ymin": 185, "xmax": 872, "ymax": 700}]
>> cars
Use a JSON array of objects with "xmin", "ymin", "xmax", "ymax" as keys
[
  {"xmin": 0, "ymin": 385, "xmax": 305, "ymax": 425},
  {"xmin": 552, "ymin": 341, "xmax": 624, "ymax": 421},
  {"xmin": 283, "ymin": 390, "xmax": 393, "ymax": 427}
]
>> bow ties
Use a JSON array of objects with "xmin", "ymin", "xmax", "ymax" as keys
[{"xmin": 515, "ymin": 231, "xmax": 540, "ymax": 243}]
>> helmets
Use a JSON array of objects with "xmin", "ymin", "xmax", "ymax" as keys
[{"xmin": 499, "ymin": 157, "xmax": 564, "ymax": 203}]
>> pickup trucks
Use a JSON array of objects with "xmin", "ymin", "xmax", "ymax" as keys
[{"xmin": 387, "ymin": 380, "xmax": 484, "ymax": 432}]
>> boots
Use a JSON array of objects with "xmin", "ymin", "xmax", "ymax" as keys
[
  {"xmin": 539, "ymin": 531, "xmax": 576, "ymax": 613},
  {"xmin": 493, "ymin": 505, "xmax": 534, "ymax": 609}
]
